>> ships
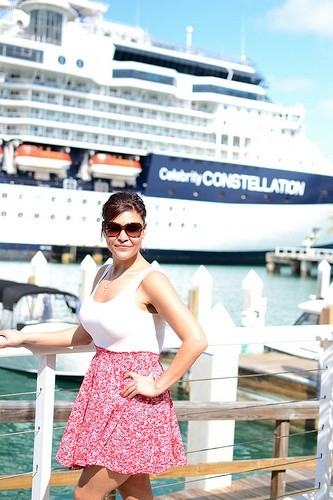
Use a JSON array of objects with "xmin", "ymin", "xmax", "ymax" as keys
[{"xmin": 0, "ymin": 0, "xmax": 333, "ymax": 267}]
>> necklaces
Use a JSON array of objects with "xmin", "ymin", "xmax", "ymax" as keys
[{"xmin": 104, "ymin": 268, "xmax": 126, "ymax": 289}]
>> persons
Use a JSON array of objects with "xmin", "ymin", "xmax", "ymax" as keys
[{"xmin": 0, "ymin": 190, "xmax": 208, "ymax": 500}]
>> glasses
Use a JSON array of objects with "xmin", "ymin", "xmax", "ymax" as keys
[{"xmin": 101, "ymin": 220, "xmax": 146, "ymax": 238}]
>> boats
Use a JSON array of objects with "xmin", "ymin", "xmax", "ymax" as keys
[
  {"xmin": 13, "ymin": 143, "xmax": 72, "ymax": 174},
  {"xmin": 87, "ymin": 152, "xmax": 142, "ymax": 181},
  {"xmin": 0, "ymin": 279, "xmax": 96, "ymax": 380},
  {"xmin": 262, "ymin": 297, "xmax": 333, "ymax": 362}
]
